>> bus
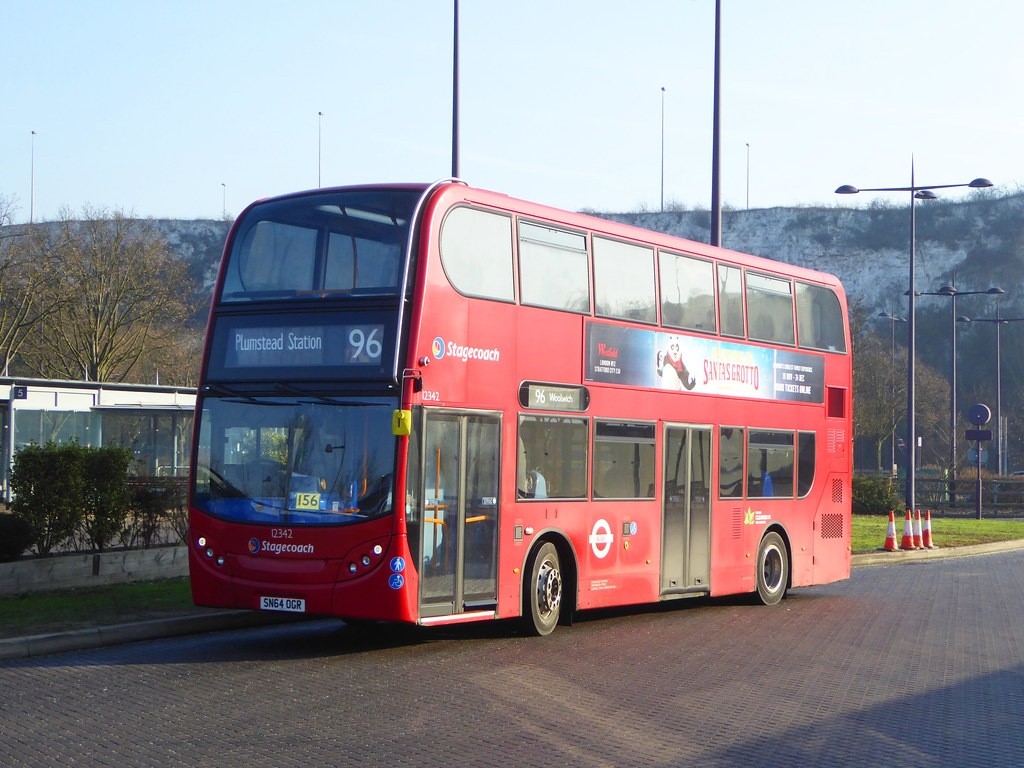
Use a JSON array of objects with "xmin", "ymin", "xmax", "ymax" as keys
[{"xmin": 188, "ymin": 176, "xmax": 855, "ymax": 638}]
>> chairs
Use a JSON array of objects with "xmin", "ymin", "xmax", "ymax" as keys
[
  {"xmin": 243, "ymin": 458, "xmax": 284, "ymax": 497},
  {"xmin": 729, "ymin": 467, "xmax": 774, "ymax": 496}
]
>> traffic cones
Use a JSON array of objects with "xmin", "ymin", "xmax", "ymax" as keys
[
  {"xmin": 921, "ymin": 508, "xmax": 937, "ymax": 550},
  {"xmin": 899, "ymin": 509, "xmax": 918, "ymax": 550},
  {"xmin": 910, "ymin": 509, "xmax": 928, "ymax": 550},
  {"xmin": 878, "ymin": 510, "xmax": 905, "ymax": 552}
]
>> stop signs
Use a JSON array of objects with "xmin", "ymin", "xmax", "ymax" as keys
[{"xmin": 897, "ymin": 439, "xmax": 906, "ymax": 451}]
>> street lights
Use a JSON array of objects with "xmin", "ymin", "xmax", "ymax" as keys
[
  {"xmin": 900, "ymin": 269, "xmax": 1006, "ymax": 509},
  {"xmin": 956, "ymin": 315, "xmax": 1024, "ymax": 476},
  {"xmin": 833, "ymin": 149, "xmax": 995, "ymax": 513},
  {"xmin": 878, "ymin": 302, "xmax": 909, "ymax": 479}
]
